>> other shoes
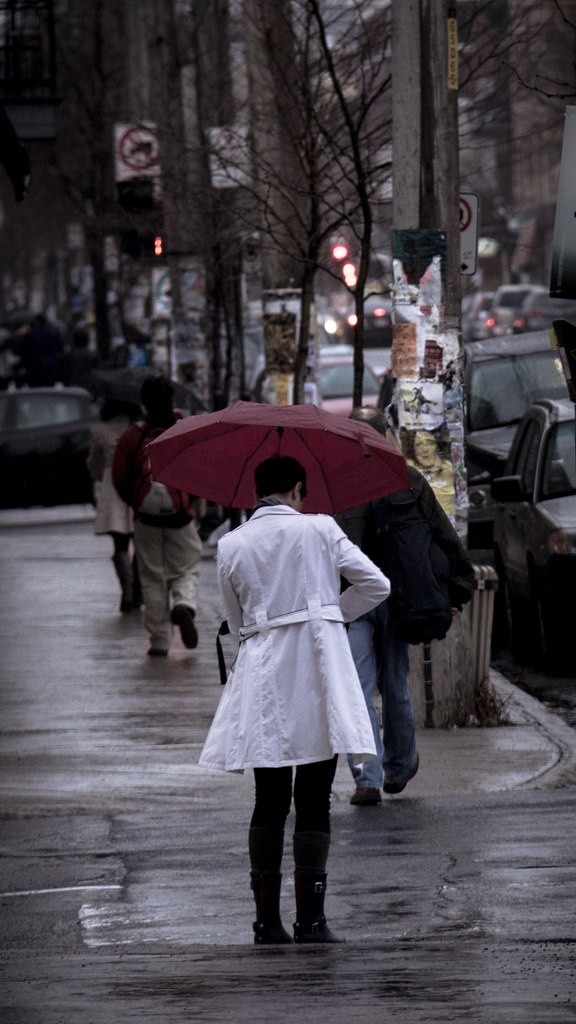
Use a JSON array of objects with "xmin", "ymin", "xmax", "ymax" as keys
[
  {"xmin": 121, "ymin": 582, "xmax": 145, "ymax": 614},
  {"xmin": 146, "ymin": 645, "xmax": 167, "ymax": 659},
  {"xmin": 179, "ymin": 614, "xmax": 197, "ymax": 647}
]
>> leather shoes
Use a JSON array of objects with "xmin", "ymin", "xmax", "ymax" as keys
[
  {"xmin": 350, "ymin": 788, "xmax": 382, "ymax": 803},
  {"xmin": 387, "ymin": 750, "xmax": 421, "ymax": 794}
]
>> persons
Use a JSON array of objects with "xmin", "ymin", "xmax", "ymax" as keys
[
  {"xmin": 198, "ymin": 455, "xmax": 376, "ymax": 944},
  {"xmin": 0, "ymin": 313, "xmax": 134, "ymax": 422},
  {"xmin": 340, "ymin": 407, "xmax": 476, "ymax": 806},
  {"xmin": 86, "ymin": 397, "xmax": 143, "ymax": 613},
  {"xmin": 112, "ymin": 375, "xmax": 202, "ymax": 658}
]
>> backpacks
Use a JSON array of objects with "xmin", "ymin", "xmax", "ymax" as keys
[
  {"xmin": 360, "ymin": 494, "xmax": 454, "ymax": 642},
  {"xmin": 131, "ymin": 415, "xmax": 192, "ymax": 517}
]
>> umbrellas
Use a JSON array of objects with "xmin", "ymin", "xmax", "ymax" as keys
[
  {"xmin": 87, "ymin": 367, "xmax": 206, "ymax": 415},
  {"xmin": 147, "ymin": 400, "xmax": 411, "ymax": 516}
]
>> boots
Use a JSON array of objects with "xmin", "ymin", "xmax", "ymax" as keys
[
  {"xmin": 250, "ymin": 868, "xmax": 292, "ymax": 943},
  {"xmin": 297, "ymin": 871, "xmax": 342, "ymax": 942}
]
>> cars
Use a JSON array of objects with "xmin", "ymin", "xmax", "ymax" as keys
[
  {"xmin": 152, "ymin": 284, "xmax": 553, "ymax": 418},
  {"xmin": 492, "ymin": 399, "xmax": 576, "ymax": 640},
  {"xmin": 381, "ymin": 330, "xmax": 575, "ymax": 570},
  {"xmin": 1, "ymin": 389, "xmax": 98, "ymax": 509}
]
